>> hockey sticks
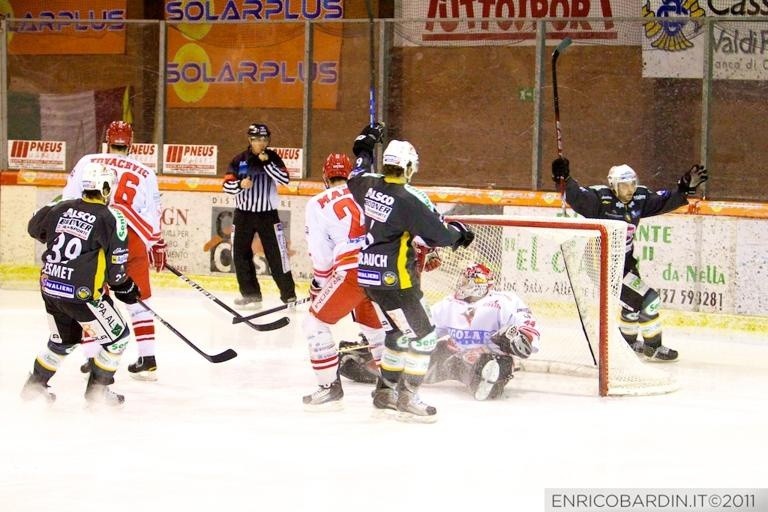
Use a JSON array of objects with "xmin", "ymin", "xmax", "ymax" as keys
[
  {"xmin": 137, "ymin": 298, "xmax": 237, "ymax": 363},
  {"xmin": 233, "ymin": 297, "xmax": 311, "ymax": 325},
  {"xmin": 551, "ymin": 37, "xmax": 572, "ymax": 217},
  {"xmin": 165, "ymin": 263, "xmax": 290, "ymax": 332},
  {"xmin": 365, "ymin": 0, "xmax": 386, "ymax": 172}
]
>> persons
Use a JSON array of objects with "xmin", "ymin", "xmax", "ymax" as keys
[
  {"xmin": 397, "ymin": 264, "xmax": 538, "ymax": 401},
  {"xmin": 553, "ymin": 156, "xmax": 709, "ymax": 363},
  {"xmin": 346, "ymin": 120, "xmax": 474, "ymax": 424},
  {"xmin": 63, "ymin": 120, "xmax": 167, "ymax": 379},
  {"xmin": 302, "ymin": 153, "xmax": 387, "ymax": 406},
  {"xmin": 19, "ymin": 168, "xmax": 141, "ymax": 407},
  {"xmin": 221, "ymin": 123, "xmax": 296, "ymax": 307}
]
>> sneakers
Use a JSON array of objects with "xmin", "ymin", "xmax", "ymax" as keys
[
  {"xmin": 373, "ymin": 377, "xmax": 398, "ymax": 411},
  {"xmin": 630, "ymin": 341, "xmax": 645, "ymax": 354},
  {"xmin": 234, "ymin": 294, "xmax": 262, "ymax": 307},
  {"xmin": 22, "ymin": 361, "xmax": 58, "ymax": 400},
  {"xmin": 643, "ymin": 344, "xmax": 679, "ymax": 361},
  {"xmin": 128, "ymin": 356, "xmax": 158, "ymax": 373},
  {"xmin": 285, "ymin": 297, "xmax": 296, "ymax": 310},
  {"xmin": 303, "ymin": 383, "xmax": 344, "ymax": 405},
  {"xmin": 85, "ymin": 360, "xmax": 124, "ymax": 407},
  {"xmin": 396, "ymin": 378, "xmax": 438, "ymax": 416}
]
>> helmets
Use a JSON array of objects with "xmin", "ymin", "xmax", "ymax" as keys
[
  {"xmin": 607, "ymin": 165, "xmax": 638, "ymax": 198},
  {"xmin": 81, "ymin": 166, "xmax": 118, "ymax": 202},
  {"xmin": 105, "ymin": 121, "xmax": 133, "ymax": 156},
  {"xmin": 455, "ymin": 263, "xmax": 493, "ymax": 301},
  {"xmin": 322, "ymin": 154, "xmax": 352, "ymax": 188},
  {"xmin": 383, "ymin": 140, "xmax": 419, "ymax": 183},
  {"xmin": 247, "ymin": 124, "xmax": 270, "ymax": 140}
]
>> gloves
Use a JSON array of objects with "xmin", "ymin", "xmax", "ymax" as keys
[
  {"xmin": 309, "ymin": 282, "xmax": 322, "ymax": 300},
  {"xmin": 501, "ymin": 327, "xmax": 529, "ymax": 358},
  {"xmin": 449, "ymin": 221, "xmax": 473, "ymax": 249},
  {"xmin": 551, "ymin": 158, "xmax": 570, "ymax": 182},
  {"xmin": 424, "ymin": 252, "xmax": 440, "ymax": 272},
  {"xmin": 110, "ymin": 279, "xmax": 141, "ymax": 304},
  {"xmin": 148, "ymin": 242, "xmax": 165, "ymax": 272},
  {"xmin": 680, "ymin": 165, "xmax": 707, "ymax": 194}
]
